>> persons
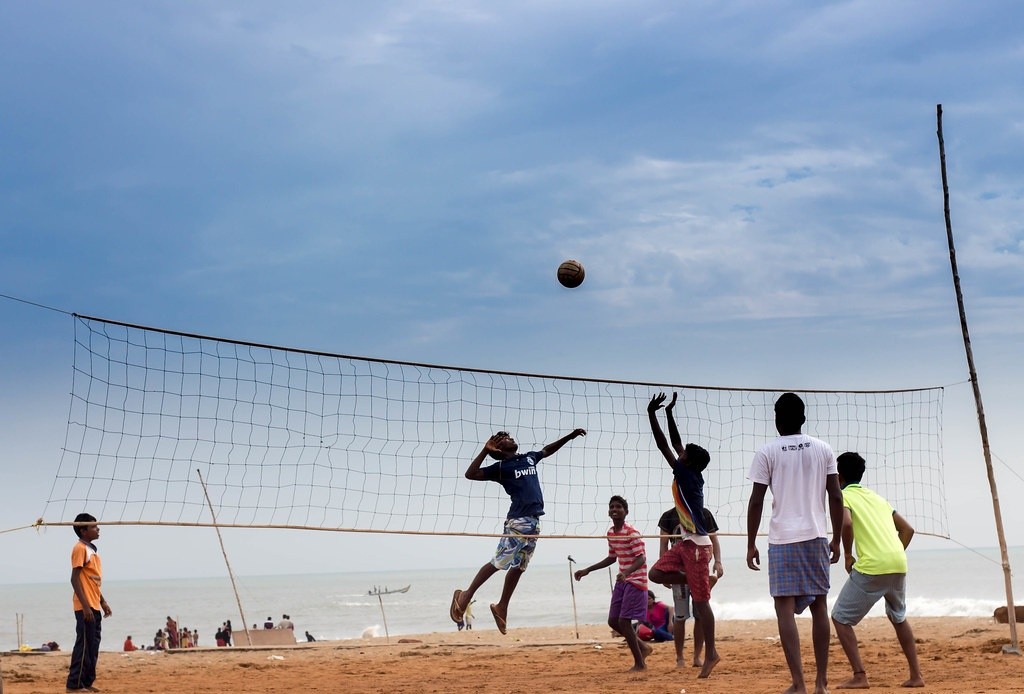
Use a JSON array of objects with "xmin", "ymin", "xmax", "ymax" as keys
[
  {"xmin": 637, "ymin": 590, "xmax": 690, "ymax": 642},
  {"xmin": 66, "ymin": 513, "xmax": 111, "ymax": 694},
  {"xmin": 747, "ymin": 392, "xmax": 842, "ymax": 694},
  {"xmin": 647, "ymin": 392, "xmax": 719, "ymax": 678},
  {"xmin": 830, "ymin": 452, "xmax": 924, "ymax": 689},
  {"xmin": 657, "ymin": 507, "xmax": 723, "ymax": 668},
  {"xmin": 451, "ymin": 428, "xmax": 587, "ymax": 636},
  {"xmin": 124, "ymin": 614, "xmax": 317, "ymax": 653},
  {"xmin": 573, "ymin": 496, "xmax": 653, "ymax": 674},
  {"xmin": 465, "ymin": 600, "xmax": 476, "ymax": 630},
  {"xmin": 457, "ymin": 620, "xmax": 464, "ymax": 631}
]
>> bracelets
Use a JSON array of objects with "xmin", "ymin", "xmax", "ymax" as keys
[{"xmin": 715, "ymin": 561, "xmax": 721, "ymax": 564}]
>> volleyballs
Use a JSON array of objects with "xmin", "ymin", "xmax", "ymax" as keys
[{"xmin": 557, "ymin": 259, "xmax": 586, "ymax": 288}]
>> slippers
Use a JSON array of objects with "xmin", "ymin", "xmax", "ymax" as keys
[
  {"xmin": 490, "ymin": 602, "xmax": 507, "ymax": 635},
  {"xmin": 451, "ymin": 590, "xmax": 468, "ymax": 623}
]
